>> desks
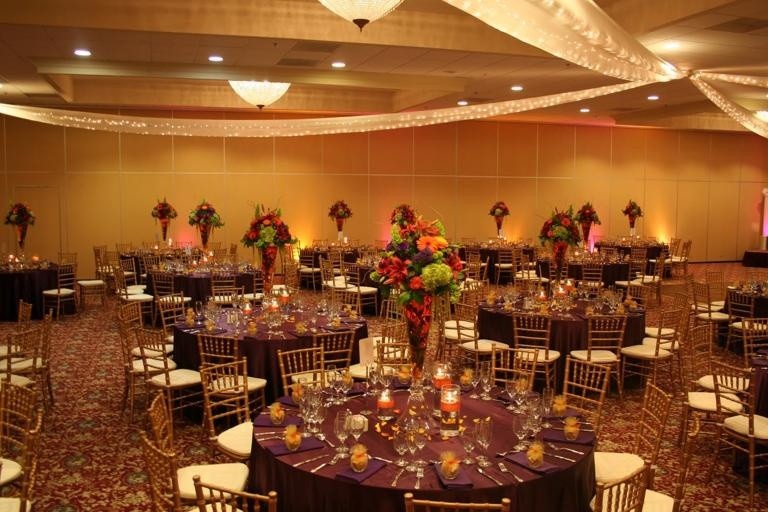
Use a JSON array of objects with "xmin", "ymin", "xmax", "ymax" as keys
[{"xmin": 253, "ymin": 377, "xmax": 597, "ymax": 510}]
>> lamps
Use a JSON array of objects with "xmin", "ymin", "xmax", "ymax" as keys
[
  {"xmin": 227, "ymin": 80, "xmax": 292, "ymax": 112},
  {"xmin": 316, "ymin": 0, "xmax": 405, "ymax": 33}
]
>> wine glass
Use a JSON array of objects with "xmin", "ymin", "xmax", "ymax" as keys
[
  {"xmin": 183, "ymin": 289, "xmax": 358, "ymax": 334},
  {"xmin": 457, "ymin": 235, "xmax": 532, "ymax": 252},
  {"xmin": 311, "ymin": 236, "xmax": 376, "ymax": 251},
  {"xmin": 156, "ymin": 257, "xmax": 253, "ymax": 276},
  {"xmin": 576, "ymin": 247, "xmax": 625, "ymax": 264},
  {"xmin": 118, "ymin": 238, "xmax": 184, "ymax": 257},
  {"xmin": 3, "ymin": 248, "xmax": 49, "ymax": 272},
  {"xmin": 595, "ymin": 234, "xmax": 658, "ymax": 248},
  {"xmin": 252, "ymin": 361, "xmax": 596, "ymax": 491},
  {"xmin": 485, "ymin": 278, "xmax": 637, "ymax": 319},
  {"xmin": 729, "ymin": 273, "xmax": 767, "ymax": 297}
]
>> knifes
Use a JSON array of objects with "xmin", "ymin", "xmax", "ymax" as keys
[{"xmin": 290, "ymin": 454, "xmax": 330, "ymax": 468}]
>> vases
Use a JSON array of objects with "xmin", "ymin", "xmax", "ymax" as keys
[
  {"xmin": 629, "ymin": 215, "xmax": 636, "ymax": 237},
  {"xmin": 494, "ymin": 216, "xmax": 504, "ymax": 238},
  {"xmin": 13, "ymin": 223, "xmax": 29, "ymax": 263},
  {"xmin": 582, "ymin": 219, "xmax": 592, "ymax": 244},
  {"xmin": 337, "ymin": 218, "xmax": 343, "ymax": 243},
  {"xmin": 198, "ymin": 223, "xmax": 212, "ymax": 254},
  {"xmin": 159, "ymin": 218, "xmax": 169, "ymax": 245}
]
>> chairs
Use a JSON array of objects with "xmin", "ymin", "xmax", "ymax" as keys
[
  {"xmin": 676, "ymin": 331, "xmax": 744, "ymax": 464},
  {"xmin": 594, "ymin": 379, "xmax": 674, "ymax": 490},
  {"xmin": 139, "ymin": 430, "xmax": 243, "ymax": 512},
  {"xmin": 563, "ymin": 354, "xmax": 611, "ymax": 438},
  {"xmin": 191, "ymin": 475, "xmax": 278, "ymax": 512},
  {"xmin": 590, "ymin": 412, "xmax": 702, "ymax": 512},
  {"xmin": 705, "ymin": 355, "xmax": 768, "ymax": 511},
  {"xmin": 594, "ymin": 455, "xmax": 653, "ymax": 511},
  {"xmin": 145, "ymin": 389, "xmax": 250, "ymax": 512},
  {"xmin": 198, "ymin": 356, "xmax": 254, "ymax": 464},
  {"xmin": 194, "ymin": 331, "xmax": 267, "ymax": 443},
  {"xmin": 0, "ymin": 250, "xmax": 82, "ymax": 512},
  {"xmin": 78, "ymin": 238, "xmax": 767, "ymax": 425},
  {"xmin": 404, "ymin": 492, "xmax": 511, "ymax": 512}
]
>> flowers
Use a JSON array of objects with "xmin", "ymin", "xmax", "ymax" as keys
[
  {"xmin": 487, "ymin": 201, "xmax": 511, "ymax": 236},
  {"xmin": 537, "ymin": 203, "xmax": 582, "ymax": 280},
  {"xmin": 186, "ymin": 198, "xmax": 225, "ymax": 244},
  {"xmin": 2, "ymin": 201, "xmax": 37, "ymax": 251},
  {"xmin": 240, "ymin": 203, "xmax": 298, "ymax": 296},
  {"xmin": 620, "ymin": 199, "xmax": 644, "ymax": 228},
  {"xmin": 370, "ymin": 203, "xmax": 478, "ymax": 374},
  {"xmin": 150, "ymin": 196, "xmax": 177, "ymax": 241},
  {"xmin": 328, "ymin": 198, "xmax": 354, "ymax": 233},
  {"xmin": 574, "ymin": 197, "xmax": 602, "ymax": 243}
]
